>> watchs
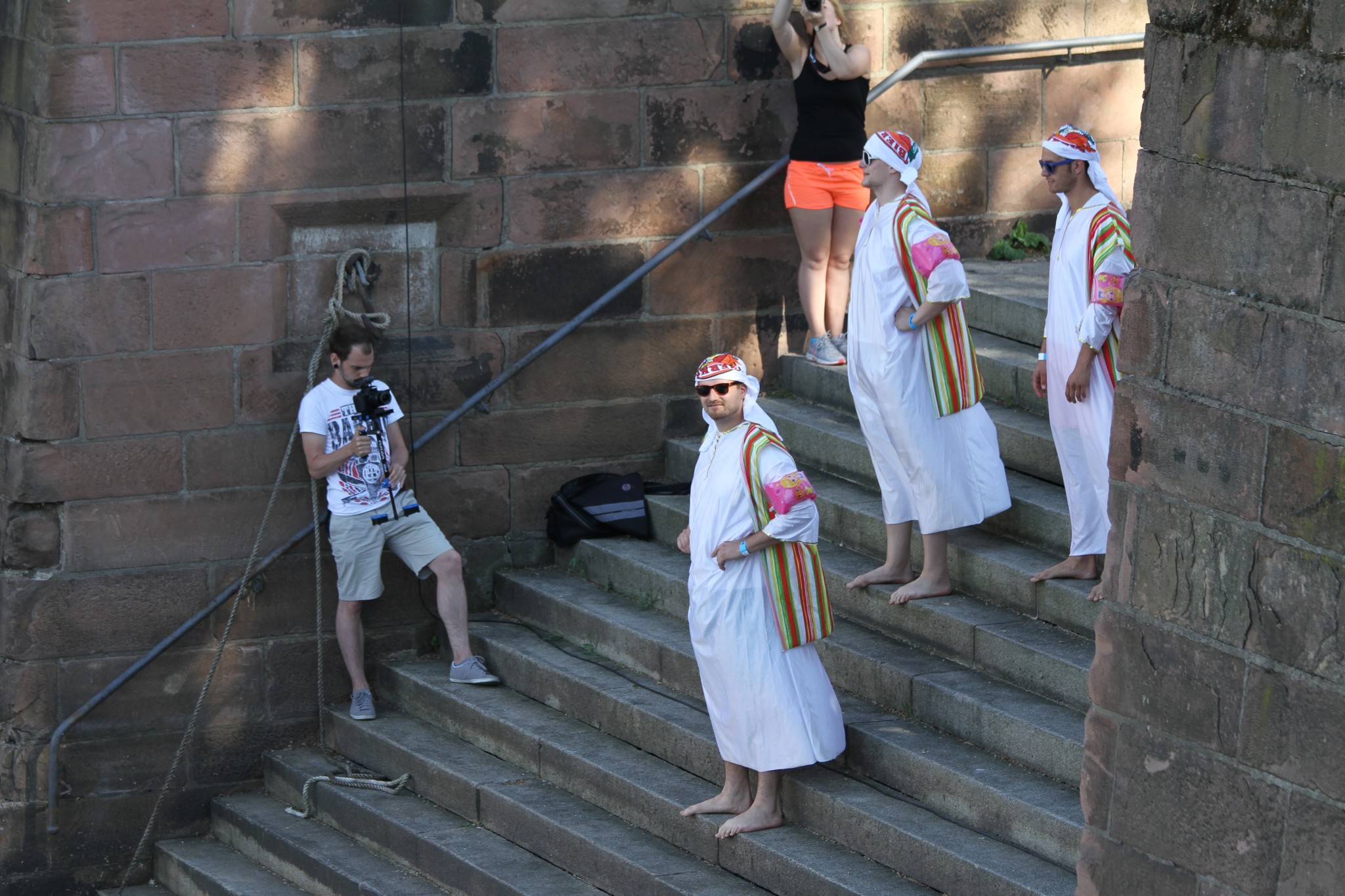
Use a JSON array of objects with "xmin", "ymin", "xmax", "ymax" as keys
[{"xmin": 813, "ymin": 22, "xmax": 827, "ymax": 31}]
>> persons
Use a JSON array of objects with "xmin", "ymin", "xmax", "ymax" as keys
[
  {"xmin": 846, "ymin": 131, "xmax": 1012, "ymax": 606},
  {"xmin": 297, "ymin": 324, "xmax": 501, "ymax": 720},
  {"xmin": 676, "ymin": 354, "xmax": 846, "ymax": 840},
  {"xmin": 770, "ymin": 0, "xmax": 872, "ymax": 366},
  {"xmin": 1029, "ymin": 125, "xmax": 1135, "ymax": 601}
]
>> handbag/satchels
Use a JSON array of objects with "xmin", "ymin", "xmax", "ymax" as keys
[{"xmin": 545, "ymin": 472, "xmax": 651, "ymax": 549}]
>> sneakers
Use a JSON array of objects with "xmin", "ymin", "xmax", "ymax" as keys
[
  {"xmin": 830, "ymin": 332, "xmax": 847, "ymax": 362},
  {"xmin": 450, "ymin": 655, "xmax": 501, "ymax": 683},
  {"xmin": 806, "ymin": 330, "xmax": 846, "ymax": 366},
  {"xmin": 349, "ymin": 688, "xmax": 376, "ymax": 719}
]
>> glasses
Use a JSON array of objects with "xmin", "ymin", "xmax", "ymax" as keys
[
  {"xmin": 1039, "ymin": 160, "xmax": 1075, "ymax": 174},
  {"xmin": 695, "ymin": 381, "xmax": 742, "ymax": 397},
  {"xmin": 862, "ymin": 151, "xmax": 880, "ymax": 166}
]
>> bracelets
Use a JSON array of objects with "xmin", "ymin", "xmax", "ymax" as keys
[
  {"xmin": 740, "ymin": 540, "xmax": 750, "ymax": 556},
  {"xmin": 1038, "ymin": 353, "xmax": 1047, "ymax": 360},
  {"xmin": 909, "ymin": 313, "xmax": 919, "ymax": 329}
]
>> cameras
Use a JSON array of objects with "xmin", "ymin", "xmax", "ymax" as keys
[
  {"xmin": 805, "ymin": 0, "xmax": 821, "ymax": 12},
  {"xmin": 352, "ymin": 376, "xmax": 392, "ymax": 413}
]
